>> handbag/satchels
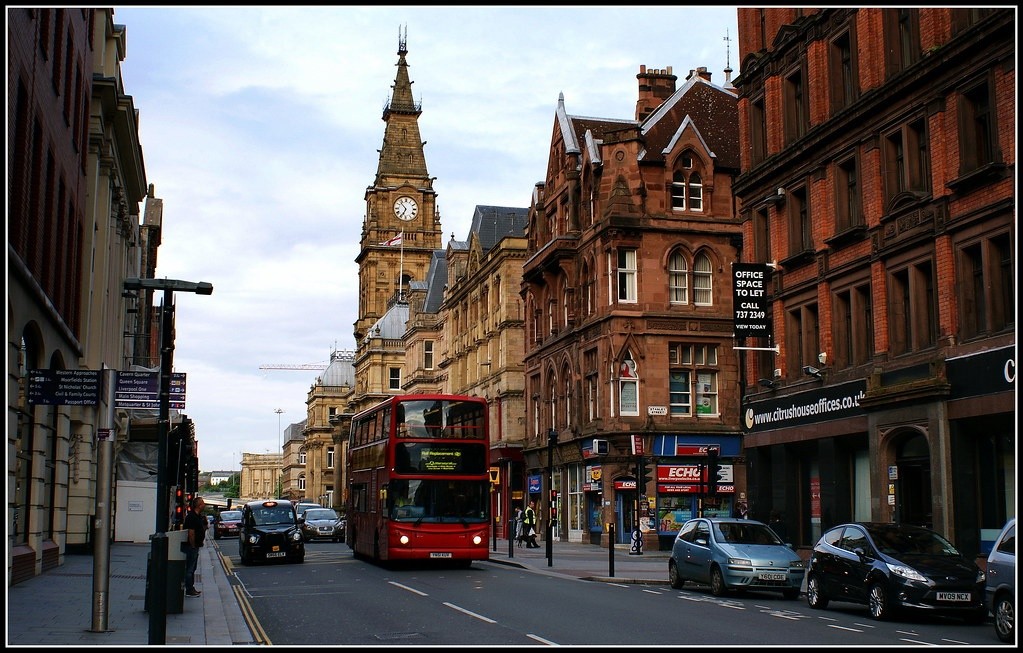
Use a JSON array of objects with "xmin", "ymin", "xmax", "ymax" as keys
[{"xmin": 529, "ymin": 527, "xmax": 535, "ymax": 536}]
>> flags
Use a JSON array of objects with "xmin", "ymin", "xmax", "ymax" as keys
[{"xmin": 383, "ymin": 232, "xmax": 402, "ymax": 246}]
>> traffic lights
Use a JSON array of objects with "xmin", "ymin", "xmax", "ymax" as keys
[
  {"xmin": 550, "ymin": 489, "xmax": 557, "ymax": 502},
  {"xmin": 640, "ymin": 456, "xmax": 652, "ymax": 492},
  {"xmin": 177, "ymin": 487, "xmax": 193, "ymax": 516},
  {"xmin": 709, "ymin": 450, "xmax": 722, "ymax": 496},
  {"xmin": 550, "ymin": 507, "xmax": 557, "ymax": 519}
]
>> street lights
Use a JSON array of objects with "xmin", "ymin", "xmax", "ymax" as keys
[
  {"xmin": 547, "ymin": 430, "xmax": 559, "ymax": 566},
  {"xmin": 126, "ymin": 279, "xmax": 213, "ymax": 642},
  {"xmin": 497, "ymin": 457, "xmax": 514, "ymax": 558}
]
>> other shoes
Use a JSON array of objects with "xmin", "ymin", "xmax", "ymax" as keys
[
  {"xmin": 526, "ymin": 544, "xmax": 540, "ymax": 548},
  {"xmin": 186, "ymin": 587, "xmax": 202, "ymax": 597}
]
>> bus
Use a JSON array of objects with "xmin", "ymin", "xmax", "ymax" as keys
[{"xmin": 346, "ymin": 396, "xmax": 492, "ymax": 569}]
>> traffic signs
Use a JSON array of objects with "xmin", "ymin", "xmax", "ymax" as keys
[{"xmin": 26, "ymin": 370, "xmax": 187, "ymax": 410}]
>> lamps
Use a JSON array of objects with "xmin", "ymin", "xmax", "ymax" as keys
[
  {"xmin": 802, "ymin": 366, "xmax": 822, "ymax": 378},
  {"xmin": 758, "ymin": 379, "xmax": 776, "ymax": 389}
]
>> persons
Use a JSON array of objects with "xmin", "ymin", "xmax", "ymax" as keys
[
  {"xmin": 183, "ymin": 497, "xmax": 208, "ymax": 597},
  {"xmin": 769, "ymin": 512, "xmax": 785, "ymax": 542},
  {"xmin": 394, "ymin": 486, "xmax": 415, "ymax": 507},
  {"xmin": 732, "ymin": 502, "xmax": 744, "ymax": 519},
  {"xmin": 514, "ymin": 501, "xmax": 541, "ymax": 549}
]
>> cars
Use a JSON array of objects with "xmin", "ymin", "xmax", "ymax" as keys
[
  {"xmin": 236, "ymin": 499, "xmax": 305, "ymax": 565},
  {"xmin": 210, "ymin": 511, "xmax": 243, "ymax": 539},
  {"xmin": 983, "ymin": 517, "xmax": 1017, "ymax": 642},
  {"xmin": 806, "ymin": 522, "xmax": 987, "ymax": 626},
  {"xmin": 258, "ymin": 510, "xmax": 292, "ymax": 521},
  {"xmin": 298, "ymin": 508, "xmax": 346, "ymax": 544},
  {"xmin": 667, "ymin": 516, "xmax": 805, "ymax": 600},
  {"xmin": 291, "ymin": 501, "xmax": 321, "ymax": 520},
  {"xmin": 207, "ymin": 516, "xmax": 215, "ymax": 522}
]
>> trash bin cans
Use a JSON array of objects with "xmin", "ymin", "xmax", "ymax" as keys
[{"xmin": 145, "ymin": 529, "xmax": 188, "ymax": 614}]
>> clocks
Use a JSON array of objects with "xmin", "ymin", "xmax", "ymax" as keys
[{"xmin": 393, "ymin": 196, "xmax": 419, "ymax": 221}]
